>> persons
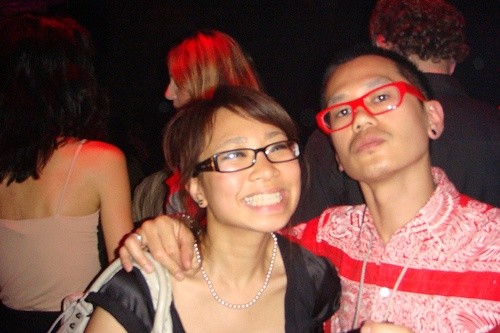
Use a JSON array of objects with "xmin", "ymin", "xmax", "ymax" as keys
[
  {"xmin": 132, "ymin": 27, "xmax": 263, "ymax": 226},
  {"xmin": 288, "ymin": 0, "xmax": 500, "ymax": 224},
  {"xmin": 118, "ymin": 47, "xmax": 500, "ymax": 333},
  {"xmin": 0, "ymin": 12, "xmax": 136, "ymax": 333},
  {"xmin": 83, "ymin": 84, "xmax": 342, "ymax": 333}
]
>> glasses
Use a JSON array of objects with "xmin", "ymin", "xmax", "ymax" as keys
[
  {"xmin": 315, "ymin": 81, "xmax": 428, "ymax": 135},
  {"xmin": 191, "ymin": 140, "xmax": 301, "ymax": 178}
]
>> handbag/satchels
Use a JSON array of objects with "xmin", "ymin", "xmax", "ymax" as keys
[{"xmin": 47, "ymin": 250, "xmax": 173, "ymax": 333}]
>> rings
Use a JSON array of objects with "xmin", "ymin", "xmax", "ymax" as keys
[{"xmin": 130, "ymin": 231, "xmax": 144, "ymax": 246}]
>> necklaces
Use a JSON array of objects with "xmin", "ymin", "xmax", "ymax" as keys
[{"xmin": 194, "ymin": 227, "xmax": 278, "ymax": 310}]
